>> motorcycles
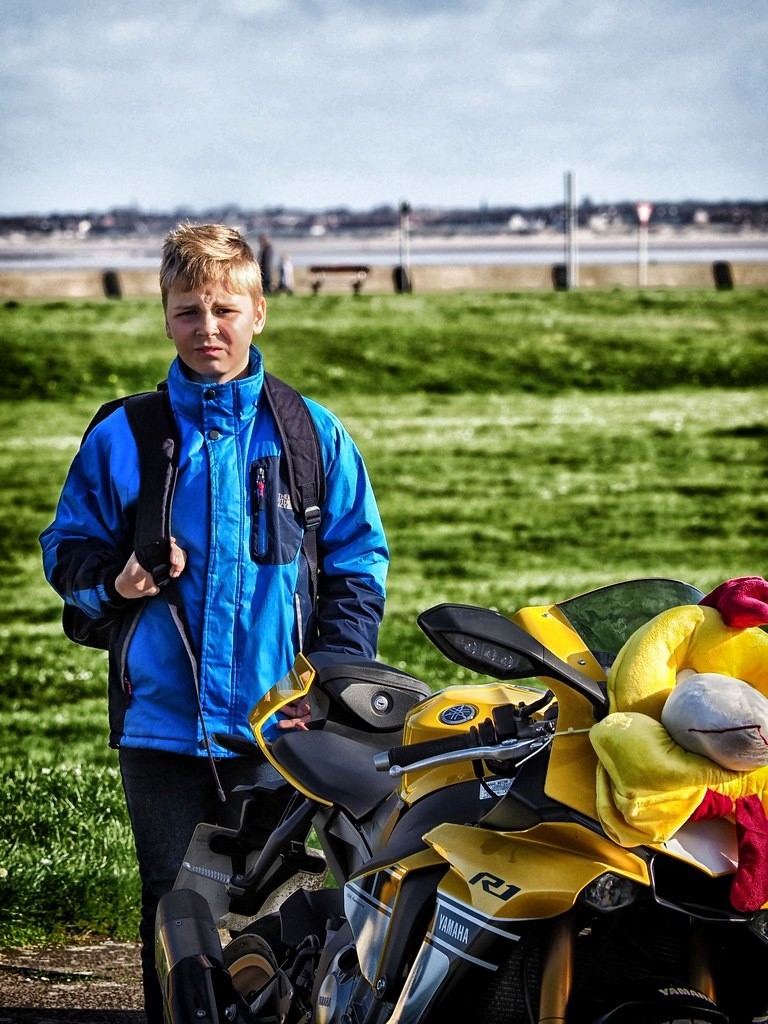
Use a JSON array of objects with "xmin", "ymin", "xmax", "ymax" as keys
[{"xmin": 150, "ymin": 579, "xmax": 768, "ymax": 1024}]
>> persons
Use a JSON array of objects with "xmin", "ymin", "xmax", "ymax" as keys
[
  {"xmin": 37, "ymin": 222, "xmax": 390, "ymax": 1024},
  {"xmin": 257, "ymin": 232, "xmax": 295, "ymax": 297}
]
